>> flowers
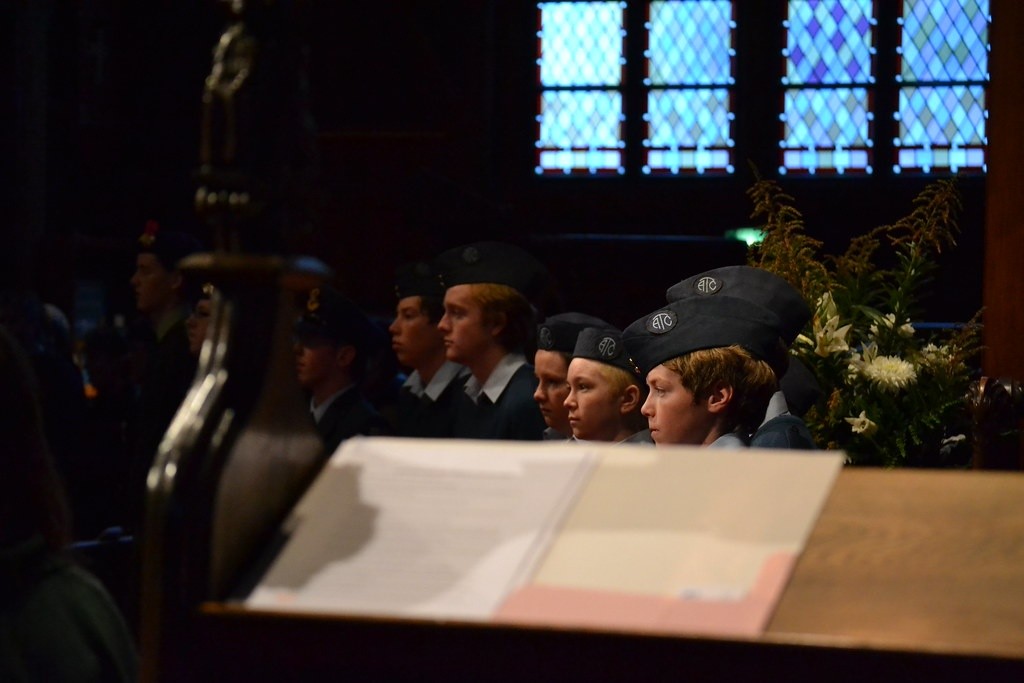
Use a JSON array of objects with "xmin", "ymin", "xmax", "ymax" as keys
[{"xmin": 746, "ymin": 171, "xmax": 990, "ymax": 467}]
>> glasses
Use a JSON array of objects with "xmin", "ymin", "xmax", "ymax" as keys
[{"xmin": 288, "ymin": 331, "xmax": 334, "ymax": 347}]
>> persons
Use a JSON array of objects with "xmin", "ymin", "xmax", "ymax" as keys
[
  {"xmin": 2, "ymin": 206, "xmax": 538, "ymax": 682},
  {"xmin": 617, "ymin": 293, "xmax": 782, "ymax": 448},
  {"xmin": 531, "ymin": 313, "xmax": 615, "ymax": 442},
  {"xmin": 559, "ymin": 327, "xmax": 656, "ymax": 447},
  {"xmin": 665, "ymin": 265, "xmax": 818, "ymax": 449}
]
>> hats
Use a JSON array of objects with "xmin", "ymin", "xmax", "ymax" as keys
[
  {"xmin": 666, "ymin": 265, "xmax": 811, "ymax": 348},
  {"xmin": 291, "ymin": 285, "xmax": 390, "ymax": 360},
  {"xmin": 135, "ymin": 224, "xmax": 203, "ymax": 272},
  {"xmin": 621, "ymin": 295, "xmax": 813, "ymax": 378},
  {"xmin": 391, "ymin": 262, "xmax": 440, "ymax": 297},
  {"xmin": 779, "ymin": 357, "xmax": 826, "ymax": 417},
  {"xmin": 538, "ymin": 312, "xmax": 617, "ymax": 353},
  {"xmin": 182, "ymin": 267, "xmax": 277, "ymax": 321},
  {"xmin": 431, "ymin": 242, "xmax": 548, "ymax": 299},
  {"xmin": 571, "ymin": 327, "xmax": 637, "ymax": 375}
]
